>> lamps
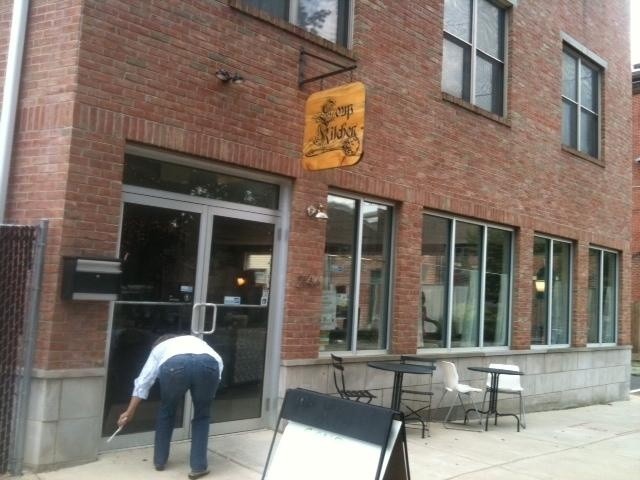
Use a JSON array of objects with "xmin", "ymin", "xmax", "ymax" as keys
[
  {"xmin": 304, "ymin": 201, "xmax": 333, "ymax": 223},
  {"xmin": 215, "ymin": 68, "xmax": 245, "ymax": 86}
]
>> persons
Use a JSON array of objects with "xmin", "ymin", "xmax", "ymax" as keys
[
  {"xmin": 421, "ymin": 291, "xmax": 441, "ymax": 338},
  {"xmin": 240, "ymin": 268, "xmax": 263, "ymax": 305},
  {"xmin": 117, "ymin": 330, "xmax": 225, "ymax": 480}
]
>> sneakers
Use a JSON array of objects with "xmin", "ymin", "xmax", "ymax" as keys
[
  {"xmin": 188, "ymin": 468, "xmax": 212, "ymax": 480},
  {"xmin": 154, "ymin": 464, "xmax": 168, "ymax": 472}
]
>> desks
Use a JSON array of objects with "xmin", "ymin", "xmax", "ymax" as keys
[
  {"xmin": 462, "ymin": 365, "xmax": 526, "ymax": 434},
  {"xmin": 367, "ymin": 359, "xmax": 438, "ymax": 440}
]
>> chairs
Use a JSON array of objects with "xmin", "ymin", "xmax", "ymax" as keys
[
  {"xmin": 435, "ymin": 360, "xmax": 487, "ymax": 430},
  {"xmin": 328, "ymin": 351, "xmax": 381, "ymax": 406},
  {"xmin": 393, "ymin": 356, "xmax": 437, "ymax": 439},
  {"xmin": 476, "ymin": 362, "xmax": 527, "ymax": 429}
]
